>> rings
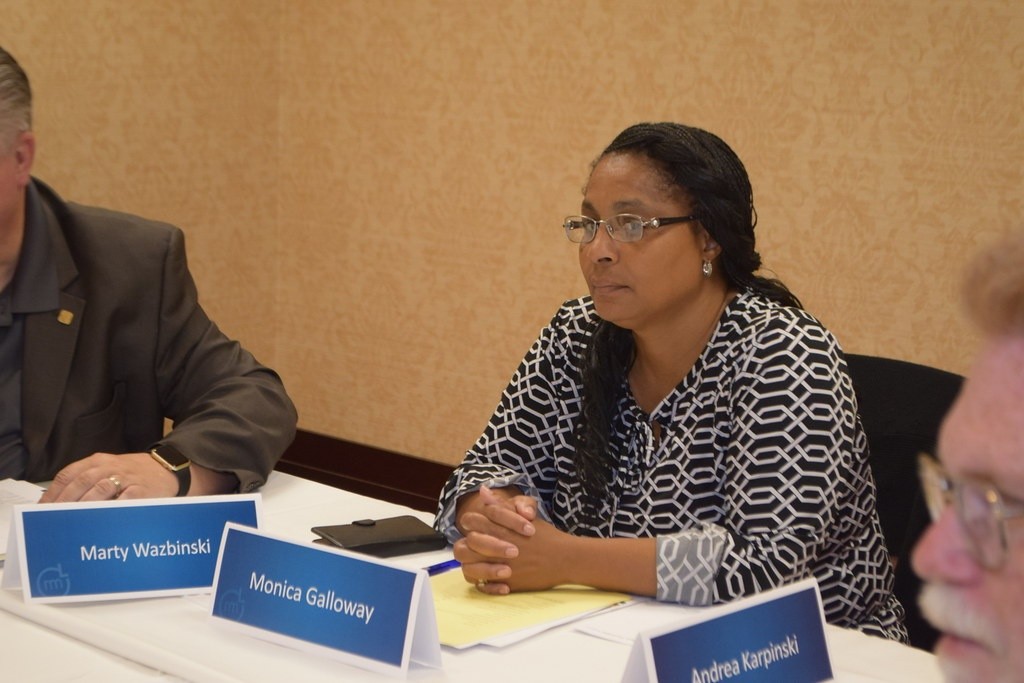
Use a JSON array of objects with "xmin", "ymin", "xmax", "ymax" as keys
[
  {"xmin": 106, "ymin": 475, "xmax": 124, "ymax": 499},
  {"xmin": 478, "ymin": 578, "xmax": 488, "ymax": 585}
]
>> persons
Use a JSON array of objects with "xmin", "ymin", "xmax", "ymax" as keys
[
  {"xmin": 439, "ymin": 122, "xmax": 908, "ymax": 646},
  {"xmin": 1, "ymin": 49, "xmax": 300, "ymax": 504},
  {"xmin": 913, "ymin": 248, "xmax": 1024, "ymax": 683}
]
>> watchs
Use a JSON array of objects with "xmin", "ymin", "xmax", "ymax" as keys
[{"xmin": 147, "ymin": 443, "xmax": 192, "ymax": 497}]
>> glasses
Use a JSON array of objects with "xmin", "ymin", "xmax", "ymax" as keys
[
  {"xmin": 919, "ymin": 447, "xmax": 1024, "ymax": 575},
  {"xmin": 562, "ymin": 211, "xmax": 697, "ymax": 245}
]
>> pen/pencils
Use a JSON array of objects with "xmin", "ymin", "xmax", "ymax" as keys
[{"xmin": 421, "ymin": 559, "xmax": 461, "ymax": 578}]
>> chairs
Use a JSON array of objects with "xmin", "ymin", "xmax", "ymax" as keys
[{"xmin": 841, "ymin": 349, "xmax": 969, "ymax": 655}]
